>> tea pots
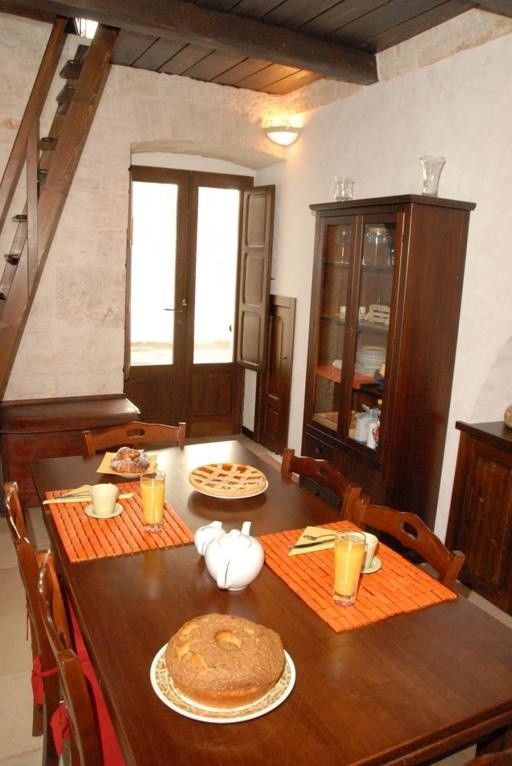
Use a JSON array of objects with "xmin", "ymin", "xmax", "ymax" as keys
[{"xmin": 194, "ymin": 519, "xmax": 267, "ymax": 594}]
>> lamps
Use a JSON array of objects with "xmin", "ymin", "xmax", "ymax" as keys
[{"xmin": 263, "ymin": 124, "xmax": 304, "ymax": 148}]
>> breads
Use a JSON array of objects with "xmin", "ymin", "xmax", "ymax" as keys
[
  {"xmin": 190, "ymin": 463, "xmax": 267, "ymax": 496},
  {"xmin": 164, "ymin": 613, "xmax": 285, "ymax": 708},
  {"xmin": 111, "ymin": 446, "xmax": 151, "ymax": 473}
]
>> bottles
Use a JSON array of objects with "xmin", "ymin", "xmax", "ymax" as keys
[
  {"xmin": 333, "ymin": 227, "xmax": 393, "ymax": 267},
  {"xmin": 355, "ymin": 399, "xmax": 382, "ymax": 449}
]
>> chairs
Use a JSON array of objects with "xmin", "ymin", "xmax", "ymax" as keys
[
  {"xmin": 457, "ymin": 745, "xmax": 512, "ymax": 766},
  {"xmin": 2, "ymin": 480, "xmax": 64, "ymax": 766},
  {"xmin": 80, "ymin": 420, "xmax": 188, "ymax": 455},
  {"xmin": 278, "ymin": 445, "xmax": 363, "ymax": 523},
  {"xmin": 348, "ymin": 491, "xmax": 466, "ymax": 591},
  {"xmin": 35, "ymin": 548, "xmax": 108, "ymax": 766}
]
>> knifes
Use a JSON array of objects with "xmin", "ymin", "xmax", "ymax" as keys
[{"xmin": 287, "ymin": 538, "xmax": 337, "ymax": 550}]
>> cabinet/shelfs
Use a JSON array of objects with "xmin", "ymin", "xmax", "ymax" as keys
[
  {"xmin": 298, "ymin": 192, "xmax": 477, "ymax": 568},
  {"xmin": 443, "ymin": 418, "xmax": 511, "ymax": 621},
  {"xmin": 1, "ymin": 392, "xmax": 142, "ymax": 518}
]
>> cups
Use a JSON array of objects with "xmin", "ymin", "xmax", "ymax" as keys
[
  {"xmin": 334, "ymin": 177, "xmax": 353, "ymax": 202},
  {"xmin": 416, "ymin": 155, "xmax": 447, "ymax": 197},
  {"xmin": 338, "ymin": 304, "xmax": 365, "ymax": 321},
  {"xmin": 91, "ymin": 483, "xmax": 118, "ymax": 514},
  {"xmin": 334, "ymin": 528, "xmax": 366, "ymax": 605},
  {"xmin": 363, "ymin": 530, "xmax": 378, "ymax": 571},
  {"xmin": 140, "ymin": 471, "xmax": 167, "ymax": 531}
]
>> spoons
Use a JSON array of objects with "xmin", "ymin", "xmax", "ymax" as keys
[{"xmin": 303, "ymin": 533, "xmax": 335, "ymax": 541}]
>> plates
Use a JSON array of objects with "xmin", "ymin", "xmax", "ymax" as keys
[
  {"xmin": 351, "ymin": 347, "xmax": 386, "ymax": 377},
  {"xmin": 187, "ymin": 469, "xmax": 269, "ymax": 498},
  {"xmin": 85, "ymin": 502, "xmax": 124, "ymax": 519},
  {"xmin": 361, "ymin": 556, "xmax": 382, "ymax": 573},
  {"xmin": 96, "ymin": 452, "xmax": 158, "ymax": 477},
  {"xmin": 149, "ymin": 640, "xmax": 298, "ymax": 725}
]
